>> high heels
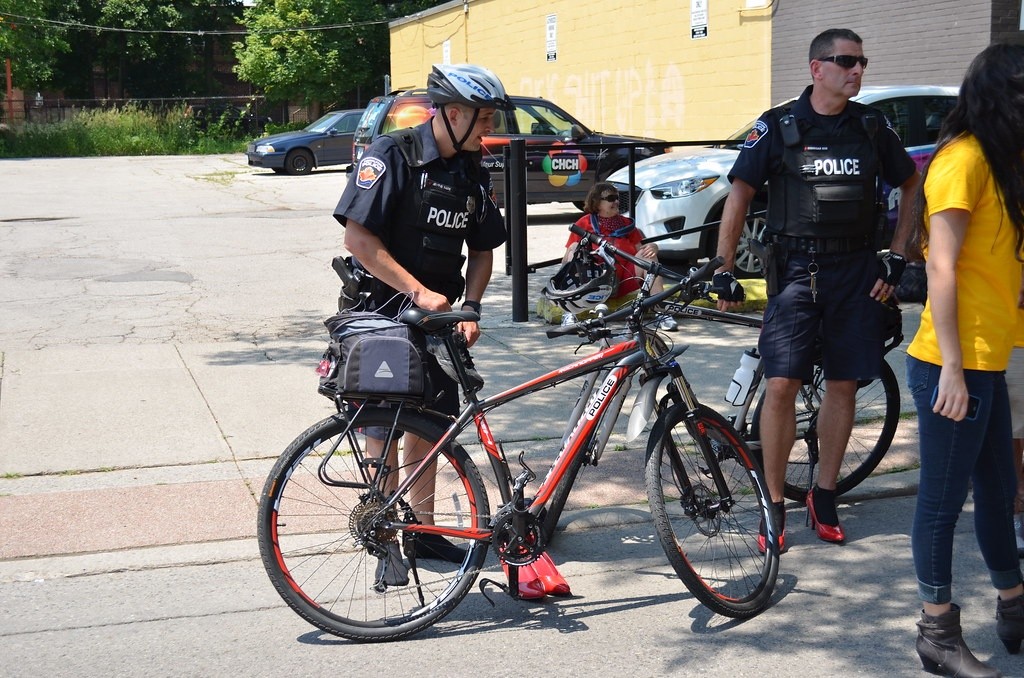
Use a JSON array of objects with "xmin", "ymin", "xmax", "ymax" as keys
[
  {"xmin": 915, "ymin": 603, "xmax": 1005, "ymax": 678},
  {"xmin": 806, "ymin": 486, "xmax": 845, "ymax": 542},
  {"xmin": 995, "ymin": 595, "xmax": 1024, "ymax": 654},
  {"xmin": 758, "ymin": 507, "xmax": 787, "ymax": 554},
  {"xmin": 499, "ymin": 529, "xmax": 570, "ymax": 600}
]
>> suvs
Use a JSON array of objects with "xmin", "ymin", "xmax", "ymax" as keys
[{"xmin": 604, "ymin": 84, "xmax": 960, "ymax": 280}]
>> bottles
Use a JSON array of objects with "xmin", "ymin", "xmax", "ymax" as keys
[{"xmin": 725, "ymin": 349, "xmax": 760, "ymax": 406}]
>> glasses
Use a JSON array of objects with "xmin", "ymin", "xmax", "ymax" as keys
[
  {"xmin": 600, "ymin": 194, "xmax": 620, "ymax": 202},
  {"xmin": 818, "ymin": 55, "xmax": 868, "ymax": 70}
]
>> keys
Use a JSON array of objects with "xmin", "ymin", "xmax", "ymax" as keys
[{"xmin": 811, "ymin": 271, "xmax": 817, "ymax": 302}]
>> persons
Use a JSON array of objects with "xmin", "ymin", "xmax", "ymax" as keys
[
  {"xmin": 713, "ymin": 28, "xmax": 921, "ymax": 554},
  {"xmin": 905, "ymin": 42, "xmax": 1024, "ymax": 678},
  {"xmin": 561, "ymin": 183, "xmax": 678, "ymax": 333},
  {"xmin": 333, "ymin": 63, "xmax": 510, "ymax": 585}
]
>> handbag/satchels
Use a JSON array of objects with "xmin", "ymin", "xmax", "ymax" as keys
[{"xmin": 324, "ymin": 291, "xmax": 427, "ymax": 396}]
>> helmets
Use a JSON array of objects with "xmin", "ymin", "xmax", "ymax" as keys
[
  {"xmin": 427, "ymin": 63, "xmax": 518, "ymax": 112},
  {"xmin": 545, "ymin": 249, "xmax": 615, "ymax": 314}
]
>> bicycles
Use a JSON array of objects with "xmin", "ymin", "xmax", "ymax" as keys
[
  {"xmin": 540, "ymin": 223, "xmax": 902, "ymax": 545},
  {"xmin": 258, "ymin": 254, "xmax": 779, "ymax": 643}
]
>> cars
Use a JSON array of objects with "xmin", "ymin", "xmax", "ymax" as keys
[
  {"xmin": 347, "ymin": 87, "xmax": 671, "ymax": 216},
  {"xmin": 183, "ymin": 104, "xmax": 273, "ymax": 137},
  {"xmin": 245, "ymin": 107, "xmax": 365, "ymax": 176}
]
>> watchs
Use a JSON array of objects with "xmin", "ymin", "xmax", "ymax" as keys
[{"xmin": 461, "ymin": 300, "xmax": 483, "ymax": 316}]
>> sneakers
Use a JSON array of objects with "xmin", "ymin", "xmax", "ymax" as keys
[
  {"xmin": 319, "ymin": 375, "xmax": 339, "ymax": 397},
  {"xmin": 425, "ymin": 329, "xmax": 485, "ymax": 389},
  {"xmin": 654, "ymin": 311, "xmax": 678, "ymax": 331},
  {"xmin": 560, "ymin": 311, "xmax": 575, "ymax": 327}
]
